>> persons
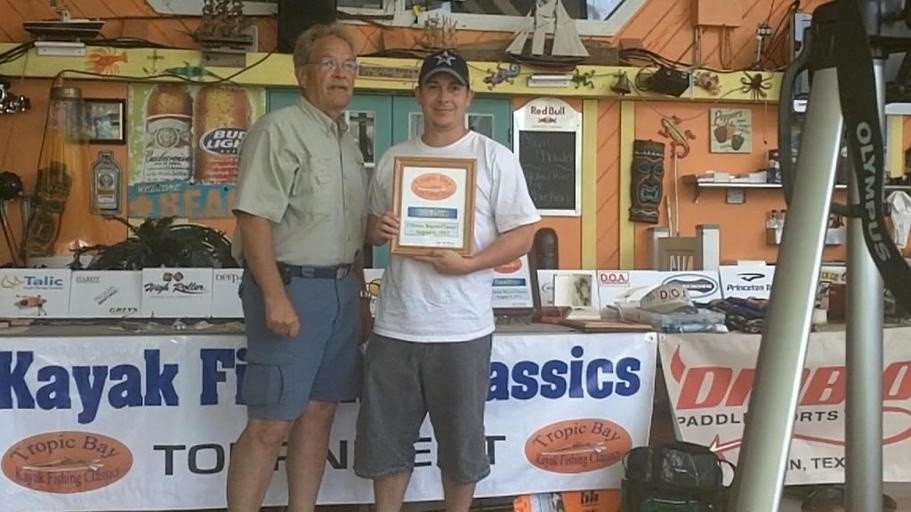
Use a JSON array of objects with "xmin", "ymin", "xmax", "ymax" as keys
[
  {"xmin": 221, "ymin": 20, "xmax": 368, "ymax": 511},
  {"xmin": 352, "ymin": 47, "xmax": 546, "ymax": 511}
]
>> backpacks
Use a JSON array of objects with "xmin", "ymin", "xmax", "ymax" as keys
[{"xmin": 620, "ymin": 443, "xmax": 737, "ymax": 510}]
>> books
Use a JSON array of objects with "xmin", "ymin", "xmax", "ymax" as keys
[{"xmin": 530, "ymin": 303, "xmax": 602, "ymax": 325}]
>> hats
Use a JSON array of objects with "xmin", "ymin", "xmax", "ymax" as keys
[
  {"xmin": 640, "ymin": 281, "xmax": 697, "ymax": 314},
  {"xmin": 418, "ymin": 49, "xmax": 471, "ymax": 93}
]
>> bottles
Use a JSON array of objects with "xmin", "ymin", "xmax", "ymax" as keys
[
  {"xmin": 192, "ymin": 83, "xmax": 253, "ymax": 184},
  {"xmin": 92, "ymin": 150, "xmax": 122, "ymax": 217},
  {"xmin": 139, "ymin": 80, "xmax": 193, "ymax": 188},
  {"xmin": 46, "ymin": 85, "xmax": 89, "ymax": 190},
  {"xmin": 767, "ymin": 208, "xmax": 785, "ymax": 228}
]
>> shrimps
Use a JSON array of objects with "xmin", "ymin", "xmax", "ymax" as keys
[{"xmin": 85, "ymin": 47, "xmax": 128, "ymax": 76}]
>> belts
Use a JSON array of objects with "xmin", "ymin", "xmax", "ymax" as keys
[{"xmin": 294, "ymin": 264, "xmax": 356, "ymax": 278}]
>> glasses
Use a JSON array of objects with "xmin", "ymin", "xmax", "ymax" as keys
[{"xmin": 295, "ymin": 56, "xmax": 361, "ymax": 76}]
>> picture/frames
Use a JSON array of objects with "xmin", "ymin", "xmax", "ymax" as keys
[
  {"xmin": 67, "ymin": 96, "xmax": 127, "ymax": 146},
  {"xmin": 512, "ymin": 97, "xmax": 583, "ymax": 218},
  {"xmin": 391, "ymin": 156, "xmax": 477, "ymax": 261},
  {"xmin": 343, "ymin": 109, "xmax": 377, "ymax": 170},
  {"xmin": 408, "ymin": 111, "xmax": 494, "ymax": 140}
]
